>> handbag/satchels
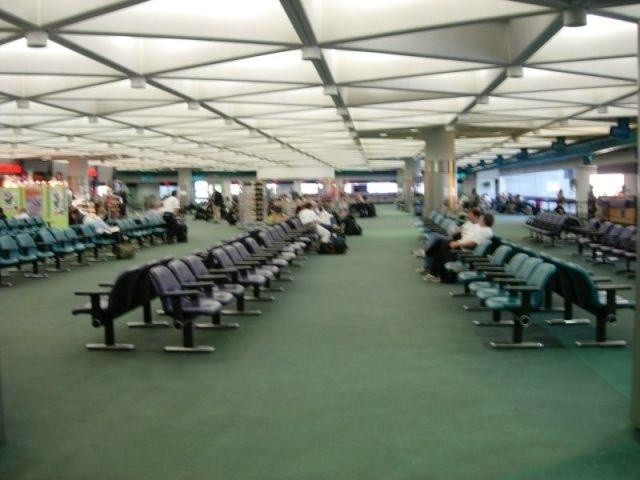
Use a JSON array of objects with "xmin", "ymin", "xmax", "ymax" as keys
[
  {"xmin": 367, "ymin": 202, "xmax": 375, "ymax": 217},
  {"xmin": 319, "ymin": 237, "xmax": 346, "ymax": 254},
  {"xmin": 113, "ymin": 243, "xmax": 135, "ymax": 259},
  {"xmin": 345, "ymin": 218, "xmax": 362, "ymax": 235}
]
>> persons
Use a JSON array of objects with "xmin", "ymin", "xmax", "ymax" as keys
[
  {"xmin": 56, "ymin": 169, "xmax": 69, "ymax": 188},
  {"xmin": 620, "ymin": 186, "xmax": 631, "ymax": 197},
  {"xmin": 458, "ymin": 189, "xmax": 525, "ymax": 215},
  {"xmin": 163, "ymin": 191, "xmax": 181, "ymax": 244},
  {"xmin": 589, "ymin": 186, "xmax": 596, "ymax": 218},
  {"xmin": 211, "ymin": 188, "xmax": 367, "ymax": 250},
  {"xmin": 423, "ymin": 214, "xmax": 494, "ymax": 283},
  {"xmin": 415, "ymin": 210, "xmax": 481, "ymax": 273},
  {"xmin": 94, "ymin": 181, "xmax": 112, "ymax": 199},
  {"xmin": 556, "ymin": 201, "xmax": 565, "ymax": 215},
  {"xmin": 558, "ymin": 190, "xmax": 565, "ymax": 206}
]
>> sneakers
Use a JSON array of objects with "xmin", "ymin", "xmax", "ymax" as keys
[
  {"xmin": 416, "ymin": 268, "xmax": 440, "ymax": 283},
  {"xmin": 412, "ymin": 248, "xmax": 427, "ymax": 259}
]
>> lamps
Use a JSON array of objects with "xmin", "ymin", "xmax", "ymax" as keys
[
  {"xmin": 301, "ymin": 45, "xmax": 351, "ymax": 119},
  {"xmin": 491, "ymin": 117, "xmax": 631, "ymax": 164},
  {"xmin": 474, "ymin": 6, "xmax": 587, "ymax": 105},
  {"xmin": 11, "ymin": 97, "xmax": 99, "ymax": 145},
  {"xmin": 23, "ymin": 28, "xmax": 49, "ymax": 49},
  {"xmin": 129, "ymin": 76, "xmax": 235, "ymax": 127}
]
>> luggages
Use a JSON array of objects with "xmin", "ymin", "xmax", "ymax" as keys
[{"xmin": 177, "ymin": 223, "xmax": 187, "ymax": 242}]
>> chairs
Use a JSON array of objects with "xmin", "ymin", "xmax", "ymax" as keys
[
  {"xmin": 1, "ymin": 212, "xmax": 187, "ymax": 284},
  {"xmin": 414, "ymin": 208, "xmax": 636, "ymax": 350},
  {"xmin": 68, "ymin": 212, "xmax": 318, "ymax": 355}
]
